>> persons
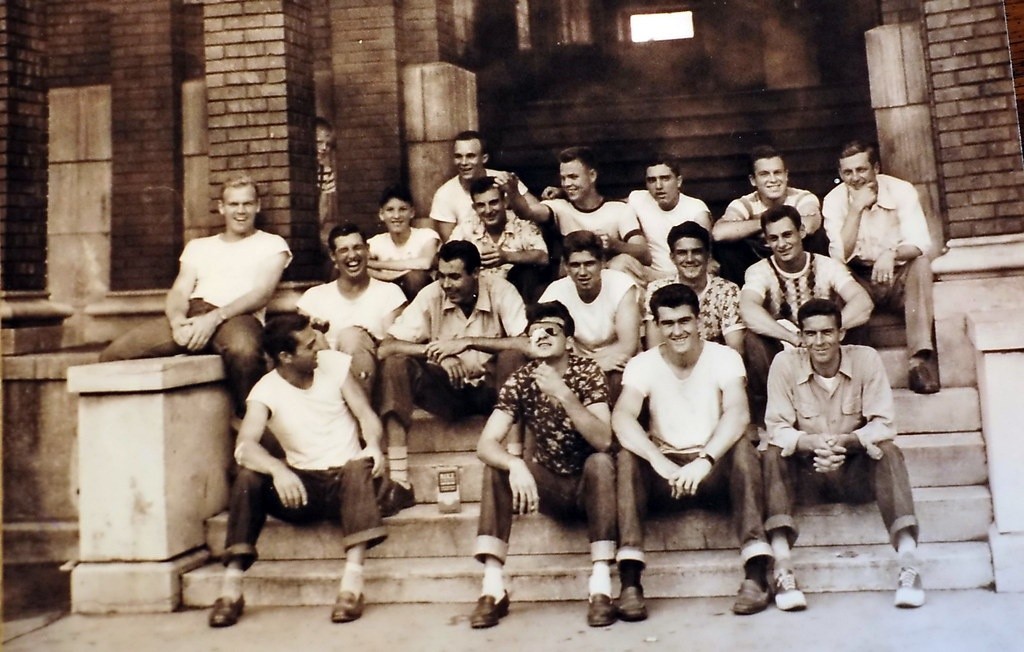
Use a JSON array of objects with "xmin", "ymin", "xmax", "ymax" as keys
[
  {"xmin": 529, "ymin": 230, "xmax": 642, "ymax": 432},
  {"xmin": 610, "ymin": 283, "xmax": 775, "ymax": 622},
  {"xmin": 711, "ymin": 145, "xmax": 822, "ymax": 268},
  {"xmin": 643, "ymin": 220, "xmax": 747, "ymax": 366},
  {"xmin": 542, "ymin": 151, "xmax": 719, "ymax": 288},
  {"xmin": 97, "ymin": 176, "xmax": 296, "ymax": 432},
  {"xmin": 379, "ymin": 239, "xmax": 532, "ymax": 517},
  {"xmin": 208, "ymin": 312, "xmax": 386, "ymax": 625},
  {"xmin": 822, "ymin": 133, "xmax": 939, "ymax": 395},
  {"xmin": 362, "ymin": 184, "xmax": 442, "ymax": 299},
  {"xmin": 468, "ymin": 300, "xmax": 618, "ymax": 626},
  {"xmin": 493, "ymin": 146, "xmax": 652, "ymax": 277},
  {"xmin": 444, "ymin": 175, "xmax": 549, "ymax": 306},
  {"xmin": 296, "ymin": 225, "xmax": 410, "ymax": 411},
  {"xmin": 762, "ymin": 298, "xmax": 927, "ymax": 611},
  {"xmin": 431, "ymin": 132, "xmax": 539, "ymax": 245},
  {"xmin": 739, "ymin": 205, "xmax": 875, "ymax": 454}
]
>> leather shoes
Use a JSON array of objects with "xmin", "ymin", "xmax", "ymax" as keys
[
  {"xmin": 210, "ymin": 593, "xmax": 244, "ymax": 626},
  {"xmin": 376, "ymin": 481, "xmax": 414, "ymax": 518},
  {"xmin": 331, "ymin": 591, "xmax": 364, "ymax": 621},
  {"xmin": 617, "ymin": 586, "xmax": 646, "ymax": 621},
  {"xmin": 909, "ymin": 365, "xmax": 939, "ymax": 393},
  {"xmin": 588, "ymin": 592, "xmax": 615, "ymax": 626},
  {"xmin": 733, "ymin": 579, "xmax": 772, "ymax": 614},
  {"xmin": 471, "ymin": 588, "xmax": 509, "ymax": 627}
]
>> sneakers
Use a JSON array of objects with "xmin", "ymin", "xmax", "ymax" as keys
[
  {"xmin": 893, "ymin": 564, "xmax": 923, "ymax": 606},
  {"xmin": 775, "ymin": 567, "xmax": 806, "ymax": 610}
]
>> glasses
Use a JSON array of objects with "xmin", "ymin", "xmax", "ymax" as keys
[{"xmin": 527, "ymin": 320, "xmax": 564, "ymax": 336}]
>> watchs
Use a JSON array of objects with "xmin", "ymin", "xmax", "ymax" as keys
[{"xmin": 698, "ymin": 451, "xmax": 715, "ymax": 466}]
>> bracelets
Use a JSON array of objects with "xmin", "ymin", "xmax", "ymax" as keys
[{"xmin": 889, "ymin": 248, "xmax": 899, "ymax": 256}]
